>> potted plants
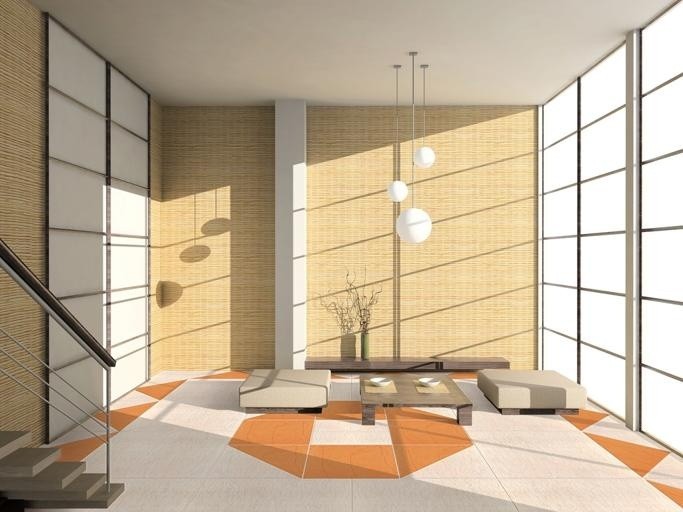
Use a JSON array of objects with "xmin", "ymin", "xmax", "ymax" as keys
[{"xmin": 346, "ymin": 267, "xmax": 382, "ymax": 360}]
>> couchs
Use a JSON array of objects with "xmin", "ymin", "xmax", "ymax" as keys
[
  {"xmin": 239, "ymin": 369, "xmax": 331, "ymax": 414},
  {"xmin": 477, "ymin": 369, "xmax": 587, "ymax": 415}
]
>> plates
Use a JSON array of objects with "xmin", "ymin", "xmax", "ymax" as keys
[
  {"xmin": 369, "ymin": 377, "xmax": 393, "ymax": 386},
  {"xmin": 417, "ymin": 377, "xmax": 440, "ymax": 387}
]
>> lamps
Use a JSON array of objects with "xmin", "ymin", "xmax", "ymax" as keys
[
  {"xmin": 415, "ymin": 64, "xmax": 435, "ymax": 169},
  {"xmin": 396, "ymin": 52, "xmax": 432, "ymax": 245},
  {"xmin": 387, "ymin": 65, "xmax": 409, "ymax": 204}
]
